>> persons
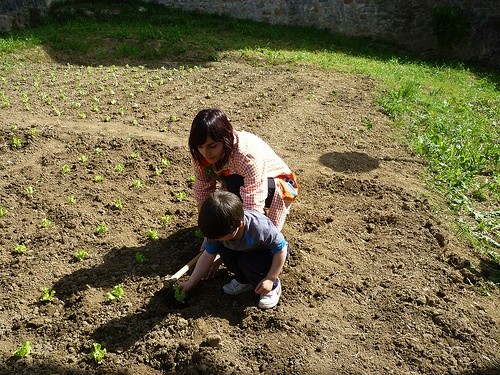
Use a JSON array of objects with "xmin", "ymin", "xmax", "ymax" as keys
[
  {"xmin": 187, "ymin": 107, "xmax": 299, "ymax": 231},
  {"xmin": 181, "ymin": 191, "xmax": 290, "ymax": 309}
]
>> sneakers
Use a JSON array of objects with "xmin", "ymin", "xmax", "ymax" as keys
[
  {"xmin": 258, "ymin": 278, "xmax": 283, "ymax": 309},
  {"xmin": 223, "ymin": 279, "xmax": 256, "ymax": 295}
]
